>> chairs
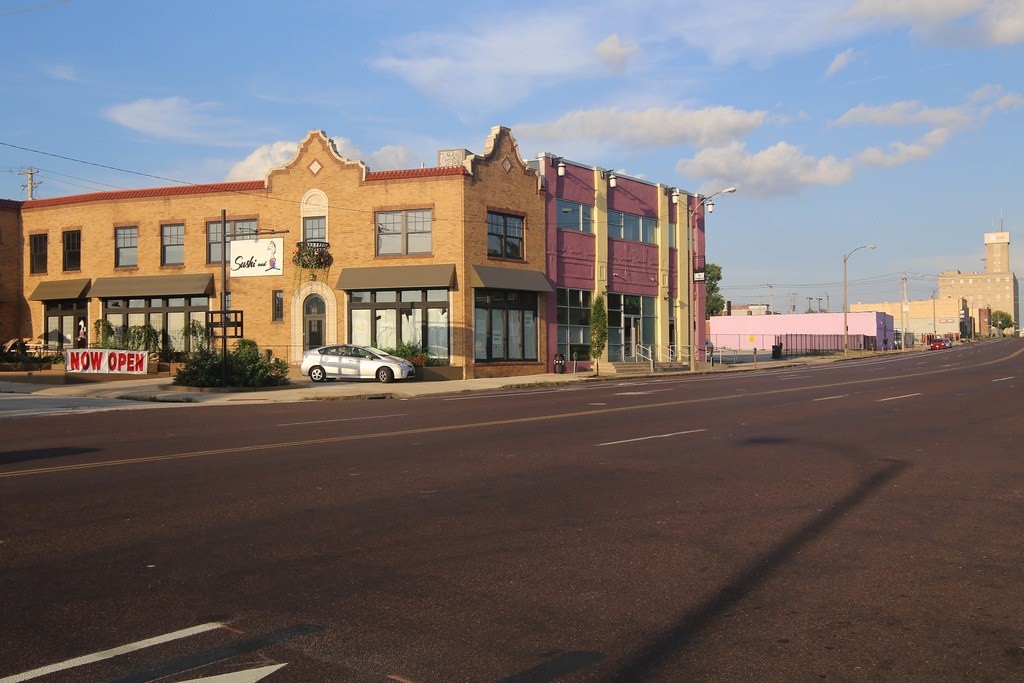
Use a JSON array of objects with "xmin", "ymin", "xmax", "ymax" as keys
[{"xmin": 353, "ymin": 348, "xmax": 360, "ymax": 356}]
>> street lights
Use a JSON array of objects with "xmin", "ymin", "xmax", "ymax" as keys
[
  {"xmin": 842, "ymin": 244, "xmax": 878, "ymax": 359},
  {"xmin": 971, "ymin": 298, "xmax": 985, "ymax": 340},
  {"xmin": 688, "ymin": 187, "xmax": 737, "ymax": 374},
  {"xmin": 933, "ymin": 284, "xmax": 952, "ymax": 339}
]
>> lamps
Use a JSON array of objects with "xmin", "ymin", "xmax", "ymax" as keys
[
  {"xmin": 601, "ymin": 169, "xmax": 617, "ymax": 188},
  {"xmin": 664, "ymin": 186, "xmax": 679, "ymax": 204},
  {"xmin": 700, "ymin": 196, "xmax": 714, "ymax": 213},
  {"xmin": 550, "ymin": 156, "xmax": 566, "ymax": 176},
  {"xmin": 310, "ymin": 274, "xmax": 315, "ymax": 281}
]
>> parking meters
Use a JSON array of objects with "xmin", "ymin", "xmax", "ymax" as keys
[
  {"xmin": 573, "ymin": 351, "xmax": 577, "ymax": 379},
  {"xmin": 753, "ymin": 348, "xmax": 758, "ymax": 369}
]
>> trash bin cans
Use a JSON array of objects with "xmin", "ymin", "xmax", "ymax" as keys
[{"xmin": 553, "ymin": 353, "xmax": 565, "ymax": 374}]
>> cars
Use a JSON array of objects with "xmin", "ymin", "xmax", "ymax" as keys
[
  {"xmin": 930, "ymin": 337, "xmax": 953, "ymax": 350},
  {"xmin": 301, "ymin": 344, "xmax": 417, "ymax": 383},
  {"xmin": 705, "ymin": 338, "xmax": 714, "ymax": 361}
]
